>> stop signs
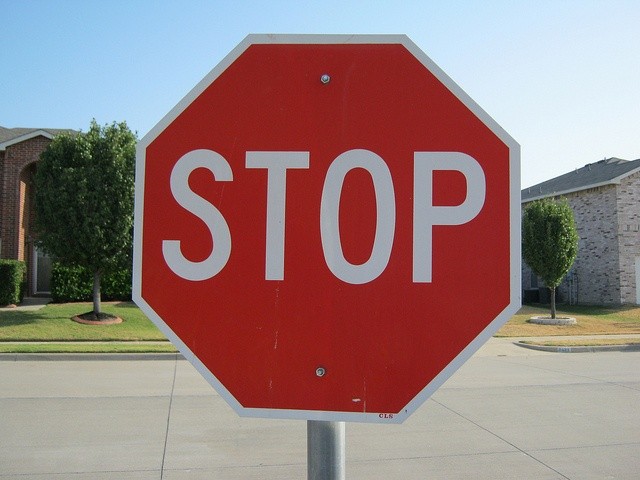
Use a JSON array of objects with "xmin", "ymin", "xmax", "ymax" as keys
[{"xmin": 132, "ymin": 33, "xmax": 520, "ymax": 426}]
